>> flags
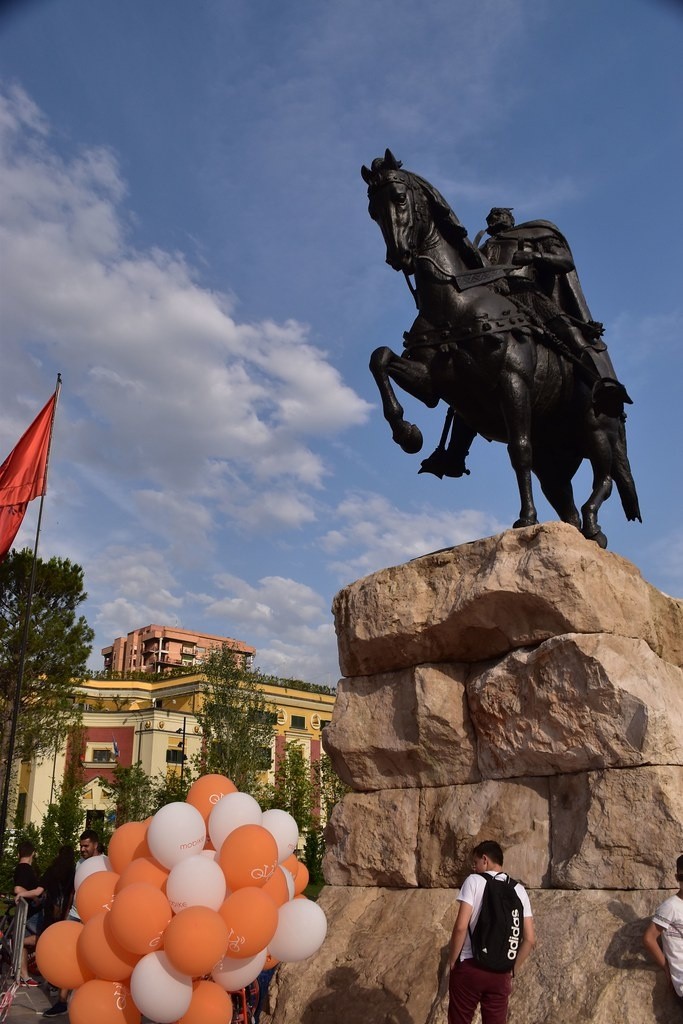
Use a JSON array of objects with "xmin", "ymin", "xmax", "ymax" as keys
[{"xmin": 0, "ymin": 382, "xmax": 62, "ymax": 561}]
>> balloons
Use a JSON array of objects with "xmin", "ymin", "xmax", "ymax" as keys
[{"xmin": 36, "ymin": 774, "xmax": 326, "ymax": 1024}]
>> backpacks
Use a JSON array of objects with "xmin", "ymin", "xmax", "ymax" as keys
[{"xmin": 467, "ymin": 872, "xmax": 523, "ymax": 972}]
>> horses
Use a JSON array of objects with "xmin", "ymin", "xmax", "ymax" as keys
[{"xmin": 360, "ymin": 148, "xmax": 645, "ymax": 551}]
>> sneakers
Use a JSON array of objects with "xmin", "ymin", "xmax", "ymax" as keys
[
  {"xmin": 43, "ymin": 1002, "xmax": 69, "ymax": 1017},
  {"xmin": 20, "ymin": 977, "xmax": 38, "ymax": 986}
]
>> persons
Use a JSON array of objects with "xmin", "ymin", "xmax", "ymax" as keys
[
  {"xmin": 421, "ymin": 208, "xmax": 643, "ymax": 523},
  {"xmin": 15, "ymin": 829, "xmax": 109, "ymax": 1017},
  {"xmin": 644, "ymin": 856, "xmax": 683, "ymax": 998},
  {"xmin": 445, "ymin": 840, "xmax": 535, "ymax": 1024}
]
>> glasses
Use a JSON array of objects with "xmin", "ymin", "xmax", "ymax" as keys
[{"xmin": 469, "ymin": 855, "xmax": 478, "ymax": 870}]
